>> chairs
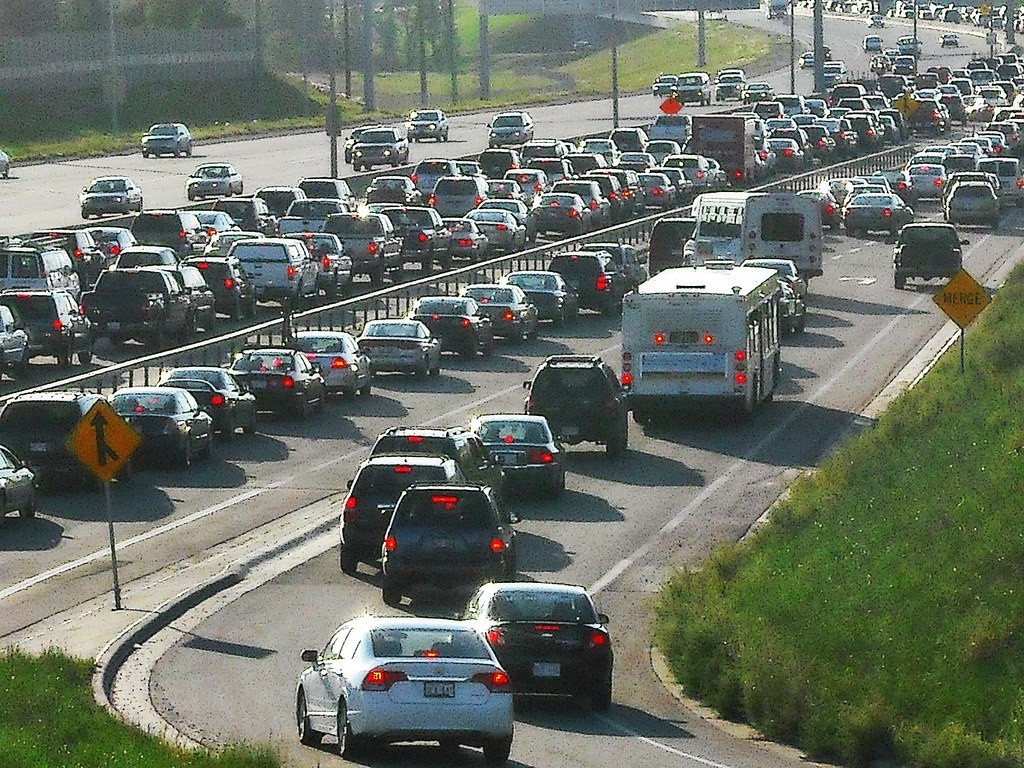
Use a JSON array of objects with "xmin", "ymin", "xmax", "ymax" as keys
[
  {"xmin": 496, "ymin": 291, "xmax": 510, "ymax": 303},
  {"xmin": 125, "ymin": 398, "xmax": 139, "ymax": 411},
  {"xmin": 374, "ymin": 641, "xmax": 402, "ymax": 657},
  {"xmin": 544, "ymin": 277, "xmax": 555, "ymax": 290},
  {"xmin": 517, "ymin": 278, "xmax": 534, "ymax": 290},
  {"xmin": 300, "ymin": 340, "xmax": 312, "ymax": 352},
  {"xmin": 171, "ymin": 375, "xmax": 189, "ymax": 388},
  {"xmin": 475, "ymin": 291, "xmax": 485, "ymax": 302},
  {"xmin": 247, "ymin": 357, "xmax": 263, "ymax": 371},
  {"xmin": 545, "ymin": 602, "xmax": 573, "ymax": 620},
  {"xmin": 525, "ymin": 427, "xmax": 542, "ymax": 443},
  {"xmin": 280, "ymin": 363, "xmax": 292, "ymax": 371},
  {"xmin": 431, "ymin": 642, "xmax": 454, "ymax": 658},
  {"xmin": 495, "ymin": 601, "xmax": 524, "ymax": 620},
  {"xmin": 333, "ymin": 343, "xmax": 341, "ymax": 353},
  {"xmin": 378, "ymin": 328, "xmax": 390, "ymax": 337},
  {"xmin": 485, "ymin": 428, "xmax": 500, "ymax": 442}
]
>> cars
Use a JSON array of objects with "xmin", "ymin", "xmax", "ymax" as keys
[
  {"xmin": 740, "ymin": 259, "xmax": 809, "ymax": 335},
  {"xmin": 651, "ymin": 75, "xmax": 677, "ymax": 98},
  {"xmin": 798, "ymin": 106, "xmax": 1024, "ymax": 290},
  {"xmin": 522, "ymin": 355, "xmax": 630, "ymax": 460},
  {"xmin": 469, "ymin": 414, "xmax": 567, "ymax": 501},
  {"xmin": 380, "ymin": 479, "xmax": 522, "ymax": 609},
  {"xmin": 451, "ymin": 580, "xmax": 613, "ymax": 710},
  {"xmin": 156, "ymin": 366, "xmax": 258, "ymax": 442},
  {"xmin": 229, "ymin": 349, "xmax": 329, "ymax": 422},
  {"xmin": 1, "ymin": 446, "xmax": 38, "ymax": 523},
  {"xmin": 295, "ymin": 616, "xmax": 515, "ymax": 766},
  {"xmin": 0, "ymin": 109, "xmax": 729, "ymax": 402},
  {"xmin": 109, "ymin": 386, "xmax": 215, "ymax": 469},
  {"xmin": 670, "ymin": 0, "xmax": 1024, "ymax": 181},
  {"xmin": 339, "ymin": 452, "xmax": 466, "ymax": 576}
]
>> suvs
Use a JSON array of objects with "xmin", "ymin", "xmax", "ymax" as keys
[
  {"xmin": 1, "ymin": 389, "xmax": 130, "ymax": 497},
  {"xmin": 368, "ymin": 424, "xmax": 511, "ymax": 510}
]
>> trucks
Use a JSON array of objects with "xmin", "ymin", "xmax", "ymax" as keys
[
  {"xmin": 683, "ymin": 192, "xmax": 825, "ymax": 295},
  {"xmin": 692, "ymin": 116, "xmax": 757, "ymax": 189}
]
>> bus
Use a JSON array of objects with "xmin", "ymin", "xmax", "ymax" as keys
[{"xmin": 622, "ymin": 261, "xmax": 783, "ymax": 425}]
